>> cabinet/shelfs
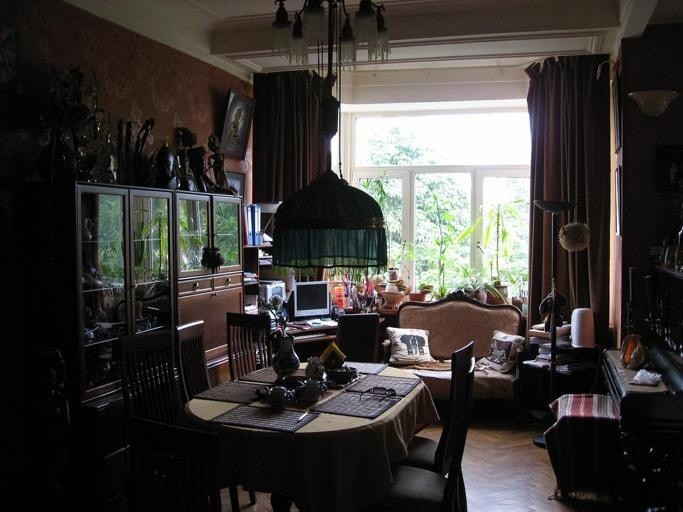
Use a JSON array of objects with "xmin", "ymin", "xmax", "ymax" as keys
[
  {"xmin": 17, "ymin": 179, "xmax": 182, "ymax": 510},
  {"xmin": 173, "ymin": 187, "xmax": 244, "ymax": 391},
  {"xmin": 241, "ymin": 201, "xmax": 289, "ymax": 297}
]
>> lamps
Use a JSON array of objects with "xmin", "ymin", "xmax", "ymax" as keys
[
  {"xmin": 271, "ymin": 0, "xmax": 394, "ymax": 73},
  {"xmin": 529, "ymin": 196, "xmax": 584, "ymax": 449},
  {"xmin": 267, "ymin": 1, "xmax": 393, "ymax": 270},
  {"xmin": 625, "ymin": 87, "xmax": 680, "ymax": 121}
]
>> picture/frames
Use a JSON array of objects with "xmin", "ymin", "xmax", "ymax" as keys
[
  {"xmin": 217, "ymin": 88, "xmax": 256, "ymax": 163},
  {"xmin": 614, "ymin": 163, "xmax": 623, "ymax": 239},
  {"xmin": 224, "ymin": 169, "xmax": 246, "ymax": 201},
  {"xmin": 609, "ymin": 68, "xmax": 622, "ymax": 158}
]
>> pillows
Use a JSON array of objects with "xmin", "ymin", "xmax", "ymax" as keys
[{"xmin": 474, "ymin": 328, "xmax": 525, "ymax": 374}]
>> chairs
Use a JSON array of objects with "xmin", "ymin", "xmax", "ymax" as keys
[
  {"xmin": 380, "ymin": 340, "xmax": 475, "ymax": 512},
  {"xmin": 333, "ymin": 313, "xmax": 381, "ymax": 363},
  {"xmin": 117, "ymin": 320, "xmax": 258, "ymax": 512},
  {"xmin": 226, "ymin": 312, "xmax": 273, "ymax": 381}
]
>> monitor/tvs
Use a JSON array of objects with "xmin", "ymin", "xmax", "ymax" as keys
[{"xmin": 294, "ymin": 281, "xmax": 330, "ymax": 319}]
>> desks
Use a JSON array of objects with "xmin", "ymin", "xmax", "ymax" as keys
[
  {"xmin": 599, "ymin": 266, "xmax": 682, "ymax": 512},
  {"xmin": 520, "ymin": 356, "xmax": 602, "ymax": 421},
  {"xmin": 185, "ymin": 359, "xmax": 441, "ymax": 512}
]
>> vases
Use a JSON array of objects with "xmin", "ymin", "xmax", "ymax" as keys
[{"xmin": 382, "ymin": 293, "xmax": 404, "ymax": 309}]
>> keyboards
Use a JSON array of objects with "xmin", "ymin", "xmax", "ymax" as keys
[{"xmin": 305, "ymin": 320, "xmax": 338, "ymax": 326}]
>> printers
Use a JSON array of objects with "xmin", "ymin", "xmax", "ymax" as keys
[{"xmin": 260, "ymin": 279, "xmax": 286, "ymax": 302}]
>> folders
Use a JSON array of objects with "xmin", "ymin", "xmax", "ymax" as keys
[{"xmin": 242, "ymin": 204, "xmax": 262, "ymax": 246}]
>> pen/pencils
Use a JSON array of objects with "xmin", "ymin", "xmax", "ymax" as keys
[{"xmin": 296, "ymin": 410, "xmax": 310, "ymax": 423}]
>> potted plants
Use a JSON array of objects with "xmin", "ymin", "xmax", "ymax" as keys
[
  {"xmin": 461, "ymin": 282, "xmax": 506, "ymax": 306},
  {"xmin": 487, "ymin": 280, "xmax": 509, "ymax": 305},
  {"xmin": 408, "ymin": 283, "xmax": 433, "ymax": 302}
]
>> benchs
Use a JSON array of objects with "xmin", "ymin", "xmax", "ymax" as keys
[
  {"xmin": 391, "ymin": 291, "xmax": 527, "ymax": 410},
  {"xmin": 553, "ymin": 392, "xmax": 619, "ymax": 502}
]
